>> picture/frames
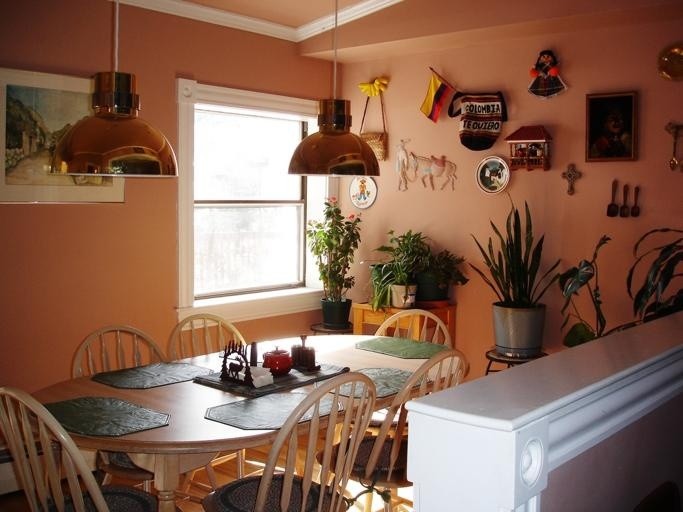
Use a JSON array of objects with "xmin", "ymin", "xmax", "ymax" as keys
[
  {"xmin": 583, "ymin": 89, "xmax": 639, "ymax": 165},
  {"xmin": 0, "ymin": 67, "xmax": 123, "ymax": 206}
]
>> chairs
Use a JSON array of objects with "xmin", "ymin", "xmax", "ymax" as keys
[
  {"xmin": 167, "ymin": 313, "xmax": 294, "ymax": 504},
  {"xmin": 376, "ymin": 307, "xmax": 452, "ymax": 346},
  {"xmin": 202, "ymin": 373, "xmax": 374, "ymax": 512},
  {"xmin": 318, "ymin": 350, "xmax": 467, "ymax": 512},
  {"xmin": 0, "ymin": 386, "xmax": 180, "ymax": 511},
  {"xmin": 72, "ymin": 321, "xmax": 187, "ymax": 500}
]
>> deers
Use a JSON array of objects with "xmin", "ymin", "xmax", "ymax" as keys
[{"xmin": 228, "ymin": 356, "xmax": 243, "ymax": 380}]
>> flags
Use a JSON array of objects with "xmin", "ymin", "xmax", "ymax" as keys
[{"xmin": 418, "ymin": 70, "xmax": 457, "ymax": 124}]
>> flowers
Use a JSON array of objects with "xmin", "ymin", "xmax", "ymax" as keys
[{"xmin": 307, "ymin": 192, "xmax": 363, "ymax": 306}]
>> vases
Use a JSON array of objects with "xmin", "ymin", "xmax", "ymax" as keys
[{"xmin": 319, "ymin": 296, "xmax": 350, "ymax": 328}]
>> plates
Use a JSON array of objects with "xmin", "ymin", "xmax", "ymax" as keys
[{"xmin": 475, "ymin": 156, "xmax": 510, "ymax": 195}]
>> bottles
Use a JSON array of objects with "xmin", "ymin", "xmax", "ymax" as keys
[
  {"xmin": 261, "ymin": 346, "xmax": 292, "ymax": 376},
  {"xmin": 291, "ymin": 334, "xmax": 315, "ymax": 369}
]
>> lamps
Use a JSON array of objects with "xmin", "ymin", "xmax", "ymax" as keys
[
  {"xmin": 47, "ymin": 1, "xmax": 178, "ymax": 180},
  {"xmin": 289, "ymin": 3, "xmax": 379, "ymax": 177}
]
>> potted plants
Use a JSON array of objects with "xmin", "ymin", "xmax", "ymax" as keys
[
  {"xmin": 467, "ymin": 198, "xmax": 561, "ymax": 356},
  {"xmin": 367, "ymin": 232, "xmax": 428, "ymax": 309},
  {"xmin": 421, "ymin": 250, "xmax": 468, "ymax": 309}
]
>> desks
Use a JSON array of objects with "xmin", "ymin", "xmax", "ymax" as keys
[
  {"xmin": 324, "ymin": 299, "xmax": 456, "ymax": 487},
  {"xmin": 482, "ymin": 350, "xmax": 548, "ymax": 376},
  {"xmin": 0, "ymin": 420, "xmax": 62, "ymax": 502},
  {"xmin": 310, "ymin": 322, "xmax": 353, "ymax": 334}
]
